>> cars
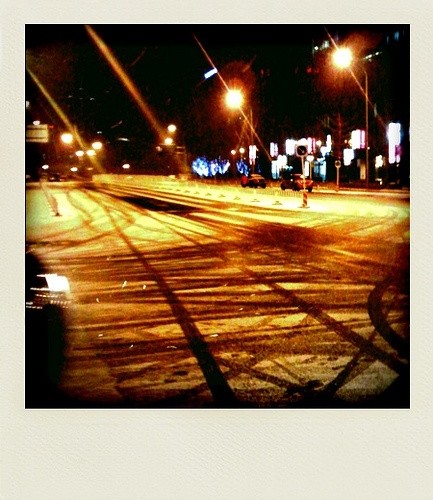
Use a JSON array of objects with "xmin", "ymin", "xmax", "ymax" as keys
[
  {"xmin": 280, "ymin": 173, "xmax": 314, "ymax": 192},
  {"xmin": 241, "ymin": 173, "xmax": 266, "ymax": 189}
]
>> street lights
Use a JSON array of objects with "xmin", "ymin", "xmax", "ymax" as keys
[
  {"xmin": 331, "ymin": 45, "xmax": 369, "ymax": 189},
  {"xmin": 224, "ymin": 84, "xmax": 253, "ymax": 176}
]
